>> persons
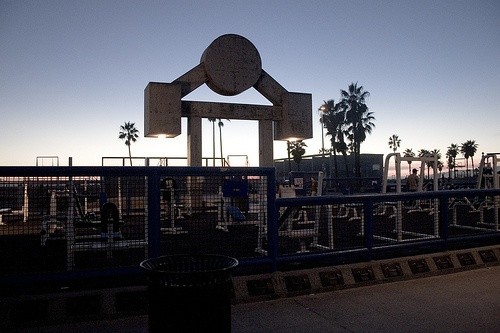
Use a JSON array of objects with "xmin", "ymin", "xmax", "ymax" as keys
[
  {"xmin": 406, "ymin": 169, "xmax": 420, "ymax": 207},
  {"xmin": 308, "ymin": 176, "xmax": 317, "ymax": 196}
]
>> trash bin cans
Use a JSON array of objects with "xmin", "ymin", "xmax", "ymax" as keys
[{"xmin": 139, "ymin": 252, "xmax": 239, "ymax": 333}]
